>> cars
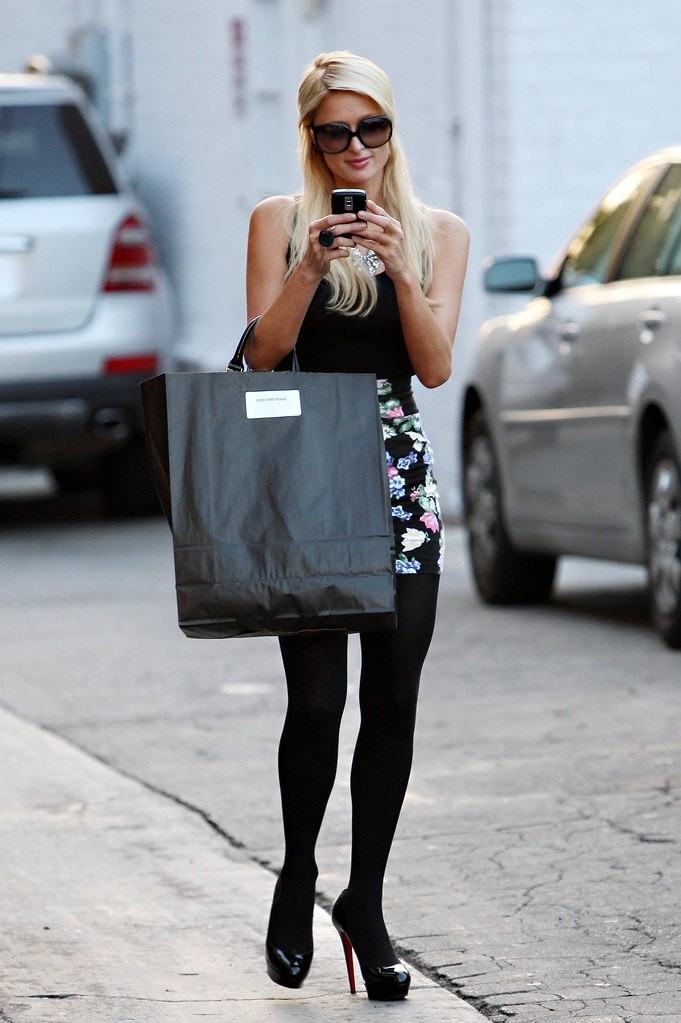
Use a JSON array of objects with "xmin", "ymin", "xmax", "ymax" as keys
[{"xmin": 451, "ymin": 137, "xmax": 681, "ymax": 648}]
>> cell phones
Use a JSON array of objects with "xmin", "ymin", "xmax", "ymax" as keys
[{"xmin": 331, "ymin": 188, "xmax": 367, "ymax": 239}]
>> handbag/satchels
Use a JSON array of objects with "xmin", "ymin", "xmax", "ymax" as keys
[{"xmin": 140, "ymin": 316, "xmax": 399, "ymax": 641}]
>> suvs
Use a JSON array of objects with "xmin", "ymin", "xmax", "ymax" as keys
[{"xmin": 0, "ymin": 56, "xmax": 175, "ymax": 525}]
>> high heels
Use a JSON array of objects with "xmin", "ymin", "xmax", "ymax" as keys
[
  {"xmin": 332, "ymin": 891, "xmax": 413, "ymax": 999},
  {"xmin": 264, "ymin": 863, "xmax": 318, "ymax": 989}
]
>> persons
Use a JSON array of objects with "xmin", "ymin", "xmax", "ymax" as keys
[{"xmin": 240, "ymin": 50, "xmax": 470, "ymax": 997}]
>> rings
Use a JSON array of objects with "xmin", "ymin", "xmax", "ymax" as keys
[{"xmin": 317, "ymin": 225, "xmax": 337, "ymax": 247}]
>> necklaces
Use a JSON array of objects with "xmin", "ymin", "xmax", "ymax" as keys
[{"xmin": 344, "ymin": 239, "xmax": 381, "ymax": 279}]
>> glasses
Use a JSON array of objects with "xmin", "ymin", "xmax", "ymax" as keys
[{"xmin": 309, "ymin": 114, "xmax": 395, "ymax": 155}]
